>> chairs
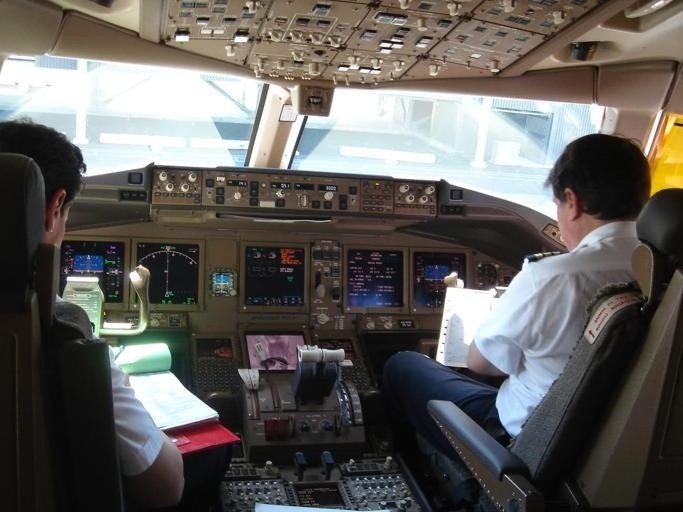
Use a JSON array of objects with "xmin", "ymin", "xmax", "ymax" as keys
[
  {"xmin": 0, "ymin": 149, "xmax": 125, "ymax": 512},
  {"xmin": 413, "ymin": 189, "xmax": 682, "ymax": 512}
]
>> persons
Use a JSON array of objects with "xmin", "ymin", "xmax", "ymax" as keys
[
  {"xmin": 0, "ymin": 119, "xmax": 184, "ymax": 512},
  {"xmin": 378, "ymin": 134, "xmax": 650, "ymax": 469}
]
